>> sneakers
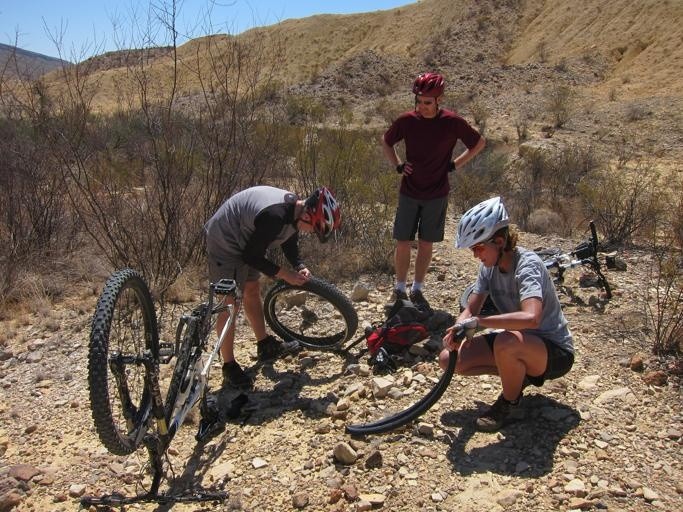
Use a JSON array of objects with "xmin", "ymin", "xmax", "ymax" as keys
[
  {"xmin": 408, "ymin": 290, "xmax": 429, "ymax": 309},
  {"xmin": 257, "ymin": 335, "xmax": 299, "ymax": 361},
  {"xmin": 476, "ymin": 374, "xmax": 530, "ymax": 432},
  {"xmin": 222, "ymin": 362, "xmax": 254, "ymax": 387},
  {"xmin": 385, "ymin": 289, "xmax": 408, "ymax": 318}
]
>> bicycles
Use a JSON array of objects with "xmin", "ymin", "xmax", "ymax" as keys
[
  {"xmin": 458, "ymin": 219, "xmax": 611, "ymax": 313},
  {"xmin": 77, "ymin": 268, "xmax": 242, "ymax": 510}
]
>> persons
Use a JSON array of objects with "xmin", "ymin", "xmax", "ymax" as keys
[
  {"xmin": 382, "ymin": 73, "xmax": 486, "ymax": 311},
  {"xmin": 203, "ymin": 184, "xmax": 340, "ymax": 387},
  {"xmin": 439, "ymin": 196, "xmax": 575, "ymax": 433}
]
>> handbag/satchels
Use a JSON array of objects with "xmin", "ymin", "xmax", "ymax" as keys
[{"xmin": 367, "ymin": 299, "xmax": 430, "ymax": 353}]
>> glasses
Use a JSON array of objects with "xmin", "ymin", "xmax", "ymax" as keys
[
  {"xmin": 469, "ymin": 238, "xmax": 493, "ymax": 253},
  {"xmin": 415, "ymin": 99, "xmax": 432, "ymax": 105}
]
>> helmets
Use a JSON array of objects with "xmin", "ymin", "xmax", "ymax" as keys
[
  {"xmin": 311, "ymin": 185, "xmax": 342, "ymax": 243},
  {"xmin": 412, "ymin": 72, "xmax": 445, "ymax": 96},
  {"xmin": 455, "ymin": 196, "xmax": 510, "ymax": 250}
]
